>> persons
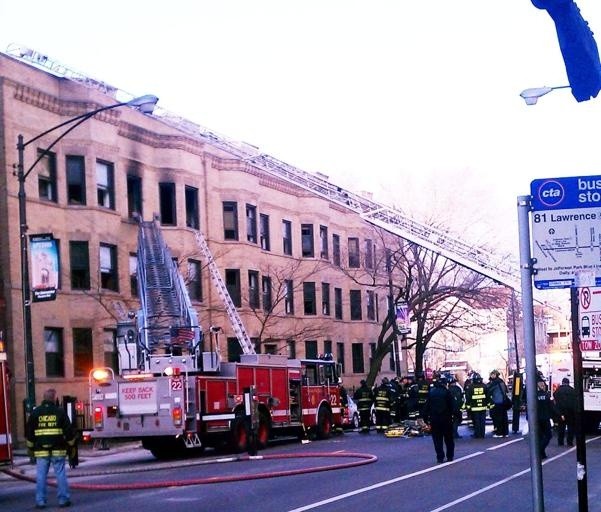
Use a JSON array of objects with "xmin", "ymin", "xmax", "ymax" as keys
[
  {"xmin": 24, "ymin": 389, "xmax": 78, "ymax": 509},
  {"xmin": 337, "ymin": 369, "xmax": 575, "ymax": 464}
]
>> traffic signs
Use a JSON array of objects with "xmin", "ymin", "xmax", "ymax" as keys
[{"xmin": 530, "ymin": 175, "xmax": 601, "ymax": 290}]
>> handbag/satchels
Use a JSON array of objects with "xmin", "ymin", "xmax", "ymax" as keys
[{"xmin": 503, "ymin": 395, "xmax": 513, "ymax": 410}]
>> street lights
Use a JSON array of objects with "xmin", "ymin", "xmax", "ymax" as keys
[
  {"xmin": 18, "ymin": 95, "xmax": 159, "ymax": 464},
  {"xmin": 520, "ymin": 86, "xmax": 570, "ymax": 105}
]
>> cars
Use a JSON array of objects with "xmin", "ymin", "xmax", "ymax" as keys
[{"xmin": 341, "ymin": 394, "xmax": 362, "ymax": 428}]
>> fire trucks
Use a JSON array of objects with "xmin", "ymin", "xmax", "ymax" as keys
[{"xmin": 82, "ymin": 212, "xmax": 343, "ymax": 461}]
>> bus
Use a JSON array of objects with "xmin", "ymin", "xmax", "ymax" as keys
[{"xmin": 522, "ymin": 352, "xmax": 601, "ymax": 431}]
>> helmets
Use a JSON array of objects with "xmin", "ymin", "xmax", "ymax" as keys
[
  {"xmin": 43, "ymin": 389, "xmax": 56, "ymax": 401},
  {"xmin": 359, "ymin": 368, "xmax": 499, "ymax": 389}
]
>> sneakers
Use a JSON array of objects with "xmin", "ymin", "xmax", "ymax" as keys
[
  {"xmin": 505, "ymin": 434, "xmax": 509, "ymax": 438},
  {"xmin": 492, "ymin": 434, "xmax": 503, "ymax": 438},
  {"xmin": 59, "ymin": 501, "xmax": 71, "ymax": 507},
  {"xmin": 36, "ymin": 505, "xmax": 46, "ymax": 509}
]
